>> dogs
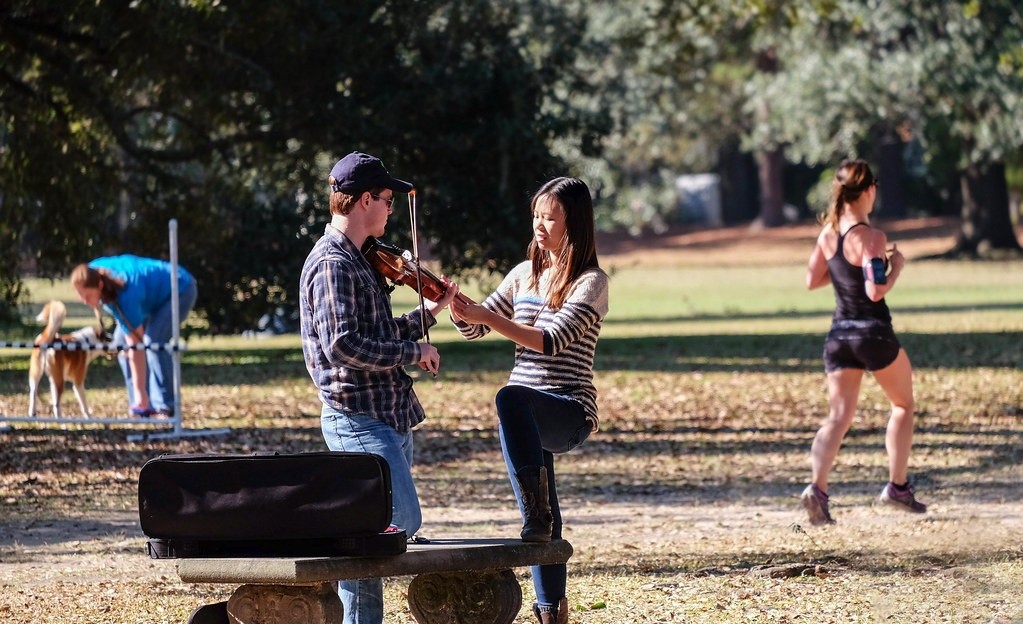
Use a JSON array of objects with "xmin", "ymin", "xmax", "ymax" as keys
[{"xmin": 29, "ymin": 299, "xmax": 115, "ymax": 419}]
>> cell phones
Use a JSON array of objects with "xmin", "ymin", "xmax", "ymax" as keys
[{"xmin": 872, "ymin": 258, "xmax": 887, "ymax": 285}]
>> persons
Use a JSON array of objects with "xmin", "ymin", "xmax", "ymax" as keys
[
  {"xmin": 70, "ymin": 254, "xmax": 198, "ymax": 423},
  {"xmin": 300, "ymin": 151, "xmax": 611, "ymax": 624},
  {"xmin": 801, "ymin": 160, "xmax": 929, "ymax": 527}
]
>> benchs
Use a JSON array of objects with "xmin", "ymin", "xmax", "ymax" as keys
[{"xmin": 175, "ymin": 536, "xmax": 576, "ymax": 624}]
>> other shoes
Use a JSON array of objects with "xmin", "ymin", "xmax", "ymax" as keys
[{"xmin": 158, "ymin": 404, "xmax": 173, "ymax": 415}]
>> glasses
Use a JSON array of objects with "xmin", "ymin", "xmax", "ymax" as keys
[
  {"xmin": 865, "ymin": 178, "xmax": 879, "ymax": 192},
  {"xmin": 358, "ymin": 192, "xmax": 395, "ymax": 209}
]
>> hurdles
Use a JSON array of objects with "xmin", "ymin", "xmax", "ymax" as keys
[{"xmin": 0, "ymin": 219, "xmax": 232, "ymax": 442}]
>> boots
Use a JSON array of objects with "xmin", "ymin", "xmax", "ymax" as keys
[
  {"xmin": 513, "ymin": 465, "xmax": 554, "ymax": 542},
  {"xmin": 533, "ymin": 598, "xmax": 568, "ymax": 624}
]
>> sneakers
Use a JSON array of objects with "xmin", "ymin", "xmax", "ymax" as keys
[
  {"xmin": 800, "ymin": 483, "xmax": 837, "ymax": 527},
  {"xmin": 879, "ymin": 482, "xmax": 927, "ymax": 513}
]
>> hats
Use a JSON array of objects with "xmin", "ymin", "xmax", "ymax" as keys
[{"xmin": 328, "ymin": 151, "xmax": 413, "ymax": 194}]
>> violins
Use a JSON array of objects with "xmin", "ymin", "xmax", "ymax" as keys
[{"xmin": 362, "ymin": 235, "xmax": 467, "ymax": 312}]
